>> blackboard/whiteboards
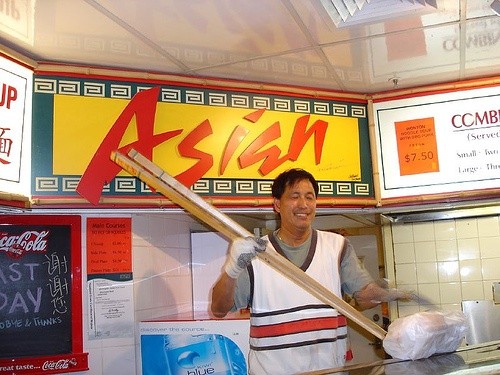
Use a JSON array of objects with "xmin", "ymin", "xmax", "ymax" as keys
[{"xmin": 0, "ymin": 213, "xmax": 89, "ymax": 374}]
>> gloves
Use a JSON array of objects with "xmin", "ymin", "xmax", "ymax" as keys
[{"xmin": 223, "ymin": 236, "xmax": 269, "ymax": 280}]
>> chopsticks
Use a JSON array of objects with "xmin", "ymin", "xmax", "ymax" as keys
[{"xmin": 111, "ymin": 149, "xmax": 387, "ymax": 340}]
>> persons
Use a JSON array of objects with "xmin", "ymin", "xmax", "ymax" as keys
[{"xmin": 210, "ymin": 168, "xmax": 421, "ymax": 375}]
